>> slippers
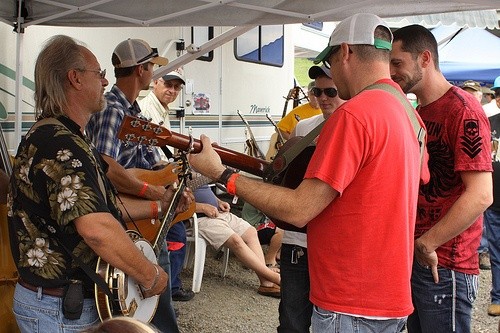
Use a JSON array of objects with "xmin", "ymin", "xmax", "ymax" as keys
[{"xmin": 266, "ymin": 258, "xmax": 281, "ymax": 273}]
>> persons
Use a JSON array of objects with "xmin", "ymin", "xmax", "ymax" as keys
[
  {"xmin": 4, "ymin": 32, "xmax": 195, "ymax": 333},
  {"xmin": 453, "ymin": 75, "xmax": 499, "ymax": 317},
  {"xmin": 271, "ymin": 61, "xmax": 357, "ymax": 333},
  {"xmin": 387, "ymin": 23, "xmax": 500, "ymax": 333},
  {"xmin": 275, "ymin": 81, "xmax": 320, "ymax": 155},
  {"xmin": 174, "ymin": 147, "xmax": 285, "ymax": 297},
  {"xmin": 184, "ymin": 13, "xmax": 432, "ymax": 332}
]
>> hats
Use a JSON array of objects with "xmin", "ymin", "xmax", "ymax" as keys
[
  {"xmin": 307, "ymin": 81, "xmax": 316, "ymax": 92},
  {"xmin": 490, "ymin": 77, "xmax": 500, "ymax": 91},
  {"xmin": 313, "ymin": 13, "xmax": 394, "ymax": 64},
  {"xmin": 162, "ymin": 66, "xmax": 186, "ymax": 85},
  {"xmin": 460, "ymin": 80, "xmax": 483, "ymax": 92},
  {"xmin": 111, "ymin": 38, "xmax": 168, "ymax": 69},
  {"xmin": 308, "ymin": 62, "xmax": 332, "ymax": 79}
]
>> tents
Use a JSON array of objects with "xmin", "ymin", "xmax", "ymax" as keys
[{"xmin": 437, "ymin": 22, "xmax": 500, "ymax": 86}]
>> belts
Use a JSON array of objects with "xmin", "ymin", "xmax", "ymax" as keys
[{"xmin": 18, "ymin": 276, "xmax": 94, "ymax": 300}]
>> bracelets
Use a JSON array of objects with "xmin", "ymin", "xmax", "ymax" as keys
[
  {"xmin": 226, "ymin": 173, "xmax": 240, "ymax": 195},
  {"xmin": 217, "ymin": 169, "xmax": 235, "ymax": 188},
  {"xmin": 157, "ymin": 200, "xmax": 162, "ymax": 220},
  {"xmin": 152, "ymin": 200, "xmax": 158, "ymax": 219},
  {"xmin": 138, "ymin": 182, "xmax": 148, "ymax": 198}
]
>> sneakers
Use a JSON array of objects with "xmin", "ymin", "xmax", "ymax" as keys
[
  {"xmin": 479, "ymin": 251, "xmax": 491, "ymax": 270},
  {"xmin": 171, "ymin": 287, "xmax": 194, "ymax": 301}
]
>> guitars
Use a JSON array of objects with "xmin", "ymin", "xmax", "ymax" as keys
[
  {"xmin": 237, "ymin": 109, "xmax": 265, "ymax": 161},
  {"xmin": 95, "ymin": 166, "xmax": 193, "ymax": 325},
  {"xmin": 266, "ymin": 114, "xmax": 286, "ymax": 149},
  {"xmin": 115, "ymin": 113, "xmax": 317, "ymax": 233},
  {"xmin": 265, "ymin": 89, "xmax": 296, "ymax": 162},
  {"xmin": 118, "ymin": 159, "xmax": 241, "ymax": 245}
]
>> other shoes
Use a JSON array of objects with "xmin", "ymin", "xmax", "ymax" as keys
[
  {"xmin": 257, "ymin": 283, "xmax": 280, "ymax": 297},
  {"xmin": 487, "ymin": 303, "xmax": 500, "ymax": 315}
]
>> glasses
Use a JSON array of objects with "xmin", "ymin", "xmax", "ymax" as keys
[
  {"xmin": 311, "ymin": 87, "xmax": 338, "ymax": 98},
  {"xmin": 136, "ymin": 48, "xmax": 159, "ymax": 64},
  {"xmin": 323, "ymin": 45, "xmax": 353, "ymax": 69},
  {"xmin": 158, "ymin": 80, "xmax": 183, "ymax": 91},
  {"xmin": 75, "ymin": 69, "xmax": 106, "ymax": 79}
]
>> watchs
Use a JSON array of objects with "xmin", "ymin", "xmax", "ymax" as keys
[{"xmin": 139, "ymin": 263, "xmax": 160, "ymax": 292}]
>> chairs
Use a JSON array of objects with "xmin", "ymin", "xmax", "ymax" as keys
[{"xmin": 185, "ymin": 213, "xmax": 229, "ymax": 292}]
